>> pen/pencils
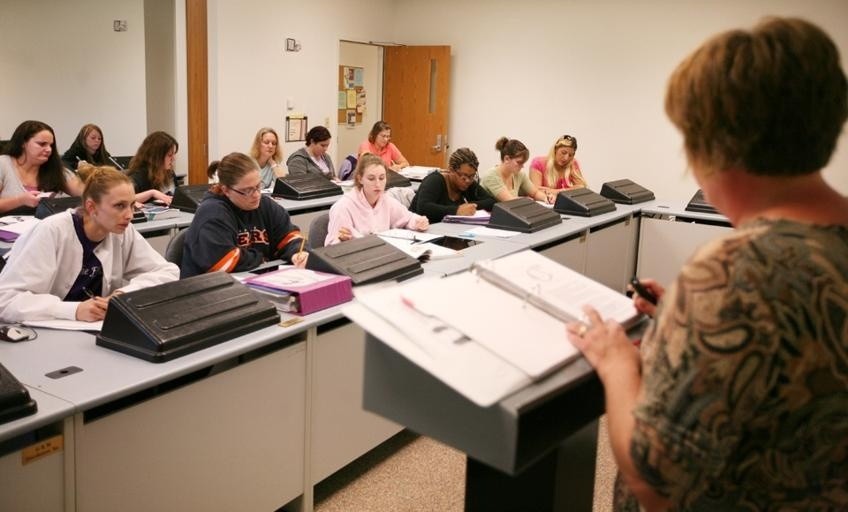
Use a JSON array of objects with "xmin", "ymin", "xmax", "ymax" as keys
[
  {"xmin": 629, "ymin": 277, "xmax": 658, "ymax": 304},
  {"xmin": 294, "ymin": 238, "xmax": 305, "ymax": 269},
  {"xmin": 82, "ymin": 287, "xmax": 97, "ymax": 300},
  {"xmin": 391, "ymin": 160, "xmax": 396, "ymax": 165},
  {"xmin": 463, "ymin": 198, "xmax": 469, "ymax": 205},
  {"xmin": 76, "ymin": 156, "xmax": 81, "ymax": 161},
  {"xmin": 544, "ymin": 190, "xmax": 551, "ymax": 203}
]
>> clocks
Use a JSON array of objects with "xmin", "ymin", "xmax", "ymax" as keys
[{"xmin": 286, "ymin": 37, "xmax": 296, "ymax": 52}]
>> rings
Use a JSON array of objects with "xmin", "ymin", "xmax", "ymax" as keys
[{"xmin": 578, "ymin": 324, "xmax": 588, "ymax": 337}]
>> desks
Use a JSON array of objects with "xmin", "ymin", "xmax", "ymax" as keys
[
  {"xmin": 427, "ymin": 216, "xmax": 586, "ymax": 288},
  {"xmin": 634, "ymin": 198, "xmax": 738, "ymax": 293},
  {"xmin": 159, "ymin": 207, "xmax": 195, "ymax": 231},
  {"xmin": 248, "ymin": 227, "xmax": 468, "ymax": 512},
  {"xmin": 409, "ymin": 180, "xmax": 423, "ymax": 194},
  {"xmin": 1, "ymin": 211, "xmax": 40, "ymax": 269},
  {"xmin": 130, "ymin": 195, "xmax": 174, "ymax": 256},
  {"xmin": 267, "ymin": 186, "xmax": 352, "ymax": 240},
  {"xmin": 0, "ymin": 301, "xmax": 312, "ymax": 512},
  {"xmin": 466, "ymin": 189, "xmax": 638, "ymax": 298},
  {"xmin": 0, "ymin": 379, "xmax": 76, "ymax": 512}
]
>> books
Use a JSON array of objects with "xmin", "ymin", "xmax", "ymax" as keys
[
  {"xmin": 443, "ymin": 208, "xmax": 491, "ymax": 225},
  {"xmin": 400, "ymin": 166, "xmax": 439, "ymax": 182},
  {"xmin": 402, "ymin": 249, "xmax": 643, "ymax": 384},
  {"xmin": 143, "ymin": 208, "xmax": 183, "ymax": 222},
  {"xmin": 242, "ymin": 281, "xmax": 298, "ymax": 312},
  {"xmin": 330, "ymin": 179, "xmax": 354, "ymax": 187},
  {"xmin": 180, "ymin": 152, "xmax": 312, "ymax": 280},
  {"xmin": 377, "ymin": 227, "xmax": 441, "ymax": 244}
]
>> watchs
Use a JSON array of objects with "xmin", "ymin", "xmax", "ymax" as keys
[{"xmin": 271, "ymin": 163, "xmax": 279, "ymax": 168}]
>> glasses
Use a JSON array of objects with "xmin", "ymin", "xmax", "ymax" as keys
[
  {"xmin": 226, "ymin": 182, "xmax": 262, "ymax": 198},
  {"xmin": 455, "ymin": 170, "xmax": 477, "ymax": 180}
]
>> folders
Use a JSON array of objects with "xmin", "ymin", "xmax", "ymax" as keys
[
  {"xmin": 243, "ymin": 268, "xmax": 354, "ymax": 316},
  {"xmin": 341, "ymin": 249, "xmax": 650, "ymax": 408}
]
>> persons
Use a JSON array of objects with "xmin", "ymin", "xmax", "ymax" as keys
[
  {"xmin": 61, "ymin": 126, "xmax": 123, "ymax": 179},
  {"xmin": 0, "ymin": 120, "xmax": 85, "ymax": 215},
  {"xmin": 481, "ymin": 137, "xmax": 549, "ymax": 204},
  {"xmin": 246, "ymin": 128, "xmax": 288, "ymax": 191},
  {"xmin": 123, "ymin": 132, "xmax": 180, "ymax": 206},
  {"xmin": 0, "ymin": 167, "xmax": 179, "ymax": 324},
  {"xmin": 357, "ymin": 120, "xmax": 410, "ymax": 172},
  {"xmin": 325, "ymin": 153, "xmax": 428, "ymax": 247},
  {"xmin": 287, "ymin": 126, "xmax": 339, "ymax": 182},
  {"xmin": 408, "ymin": 148, "xmax": 500, "ymax": 224},
  {"xmin": 567, "ymin": 20, "xmax": 847, "ymax": 512},
  {"xmin": 529, "ymin": 136, "xmax": 586, "ymax": 198}
]
